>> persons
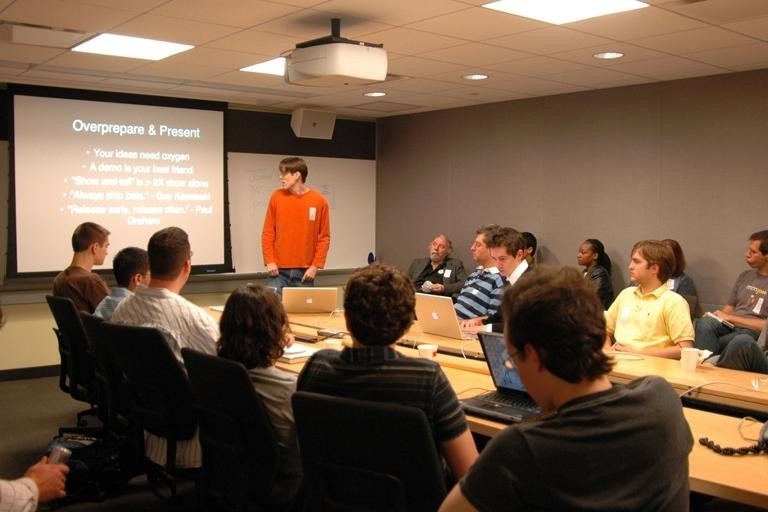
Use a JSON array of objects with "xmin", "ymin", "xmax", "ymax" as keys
[
  {"xmin": 296, "ymin": 263, "xmax": 482, "ymax": 491},
  {"xmin": 262, "ymin": 158, "xmax": 332, "ymax": 299},
  {"xmin": 53, "ymin": 222, "xmax": 110, "ymax": 314},
  {"xmin": 218, "ymin": 283, "xmax": 300, "ymax": 461},
  {"xmin": 92, "ymin": 246, "xmax": 150, "ymax": 321},
  {"xmin": 110, "ymin": 227, "xmax": 222, "ymax": 483},
  {"xmin": 1, "ymin": 454, "xmax": 163, "ymax": 512},
  {"xmin": 432, "ymin": 265, "xmax": 694, "ymax": 512}
]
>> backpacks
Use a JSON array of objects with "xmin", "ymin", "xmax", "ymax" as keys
[{"xmin": 38, "ymin": 424, "xmax": 135, "ymax": 512}]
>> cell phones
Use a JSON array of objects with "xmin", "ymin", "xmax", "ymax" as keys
[{"xmin": 47, "ymin": 446, "xmax": 73, "ymax": 464}]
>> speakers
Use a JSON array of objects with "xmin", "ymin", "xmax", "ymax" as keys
[{"xmin": 290, "ymin": 108, "xmax": 337, "ymax": 140}]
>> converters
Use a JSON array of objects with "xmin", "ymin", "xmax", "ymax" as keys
[{"xmin": 296, "ymin": 334, "xmax": 319, "ymax": 343}]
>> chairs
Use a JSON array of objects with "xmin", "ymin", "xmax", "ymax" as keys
[{"xmin": 45, "ymin": 293, "xmax": 766, "ymax": 510}]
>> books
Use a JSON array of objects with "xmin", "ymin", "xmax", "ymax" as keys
[
  {"xmin": 210, "ymin": 305, "xmax": 226, "ymax": 312},
  {"xmin": 277, "ymin": 343, "xmax": 319, "ymax": 364}
]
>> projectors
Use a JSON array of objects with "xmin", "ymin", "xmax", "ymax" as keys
[{"xmin": 283, "ymin": 42, "xmax": 387, "ymax": 87}]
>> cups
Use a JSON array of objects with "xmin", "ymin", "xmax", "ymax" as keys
[
  {"xmin": 680, "ymin": 346, "xmax": 699, "ymax": 375},
  {"xmin": 417, "ymin": 345, "xmax": 438, "ymax": 362}
]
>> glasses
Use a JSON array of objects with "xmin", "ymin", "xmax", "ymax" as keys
[{"xmin": 501, "ymin": 350, "xmax": 521, "ymax": 372}]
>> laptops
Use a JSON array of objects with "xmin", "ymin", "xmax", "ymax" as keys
[
  {"xmin": 210, "ymin": 283, "xmax": 277, "ymax": 313},
  {"xmin": 414, "ymin": 292, "xmax": 480, "ymax": 340},
  {"xmin": 456, "ymin": 330, "xmax": 545, "ymax": 423},
  {"xmin": 281, "ymin": 287, "xmax": 338, "ymax": 313}
]
